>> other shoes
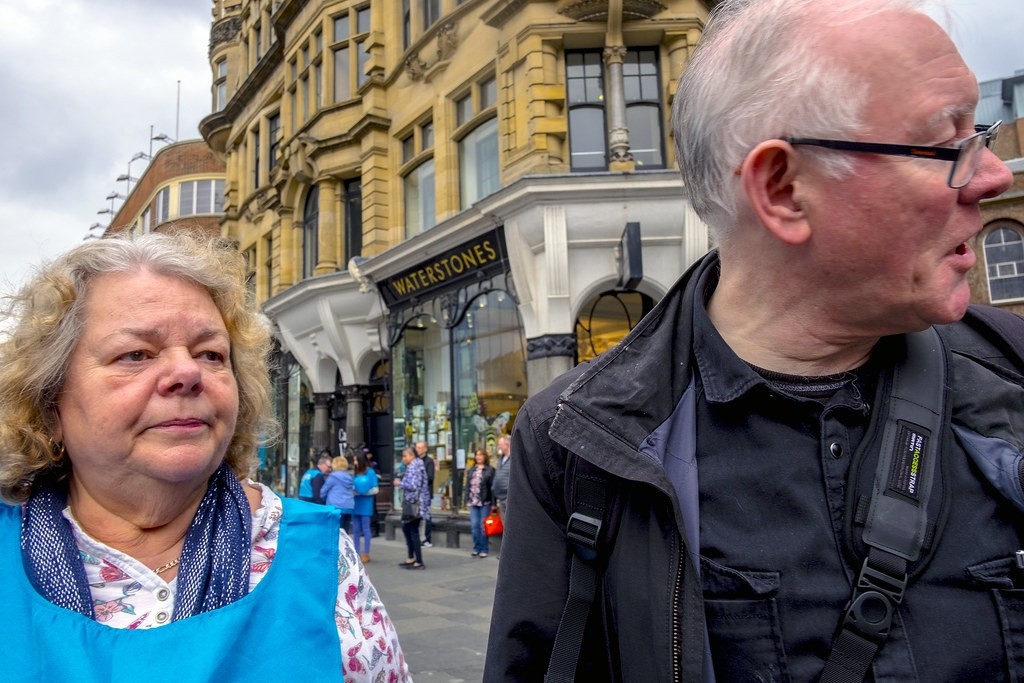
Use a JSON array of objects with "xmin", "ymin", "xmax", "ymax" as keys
[
  {"xmin": 471, "ymin": 551, "xmax": 488, "ymax": 557},
  {"xmin": 361, "ymin": 555, "xmax": 370, "ymax": 564},
  {"xmin": 399, "ymin": 561, "xmax": 425, "ymax": 570},
  {"xmin": 421, "ymin": 542, "xmax": 432, "ymax": 549}
]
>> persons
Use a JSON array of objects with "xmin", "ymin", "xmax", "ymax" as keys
[
  {"xmin": 466, "ymin": 436, "xmax": 511, "ymax": 557},
  {"xmin": 0, "ymin": 224, "xmax": 414, "ymax": 683},
  {"xmin": 482, "ymin": 0, "xmax": 1024, "ymax": 683},
  {"xmin": 393, "ymin": 441, "xmax": 434, "ymax": 571},
  {"xmin": 298, "ymin": 446, "xmax": 381, "ymax": 562}
]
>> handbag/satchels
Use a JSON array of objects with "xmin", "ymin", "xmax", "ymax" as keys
[
  {"xmin": 483, "ymin": 509, "xmax": 503, "ymax": 537},
  {"xmin": 401, "ymin": 503, "xmax": 419, "ymax": 519}
]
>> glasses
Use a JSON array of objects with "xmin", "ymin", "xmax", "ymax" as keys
[{"xmin": 735, "ymin": 119, "xmax": 1003, "ymax": 189}]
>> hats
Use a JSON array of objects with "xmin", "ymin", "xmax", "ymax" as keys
[{"xmin": 363, "ymin": 448, "xmax": 372, "ymax": 454}]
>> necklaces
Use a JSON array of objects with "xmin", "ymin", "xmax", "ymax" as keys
[{"xmin": 154, "ymin": 557, "xmax": 180, "ymax": 574}]
